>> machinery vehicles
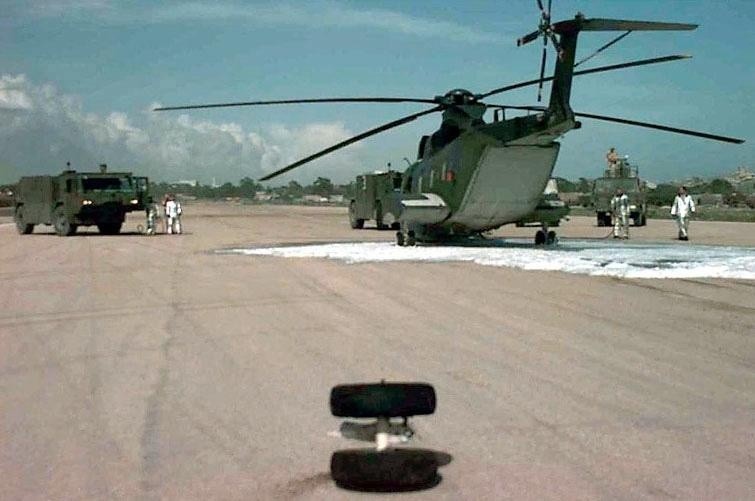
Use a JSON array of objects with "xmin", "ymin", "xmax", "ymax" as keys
[
  {"xmin": 516, "ymin": 178, "xmax": 571, "ymax": 226},
  {"xmin": 591, "ymin": 155, "xmax": 647, "ymax": 226},
  {"xmin": 12, "ymin": 161, "xmax": 149, "ymax": 236},
  {"xmin": 348, "ymin": 163, "xmax": 404, "ymax": 230}
]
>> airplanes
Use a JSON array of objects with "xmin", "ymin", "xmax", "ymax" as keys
[{"xmin": 154, "ymin": 0, "xmax": 745, "ymax": 247}]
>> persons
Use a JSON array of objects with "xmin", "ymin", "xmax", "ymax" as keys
[
  {"xmin": 146, "ymin": 197, "xmax": 160, "ymax": 235},
  {"xmin": 671, "ymin": 186, "xmax": 696, "ymax": 240},
  {"xmin": 610, "ymin": 188, "xmax": 631, "ymax": 239},
  {"xmin": 607, "ymin": 147, "xmax": 617, "ymax": 169},
  {"xmin": 165, "ymin": 194, "xmax": 182, "ymax": 235}
]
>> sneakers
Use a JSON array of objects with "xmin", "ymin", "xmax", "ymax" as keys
[
  {"xmin": 613, "ymin": 234, "xmax": 628, "ymax": 241},
  {"xmin": 679, "ymin": 234, "xmax": 689, "ymax": 242}
]
